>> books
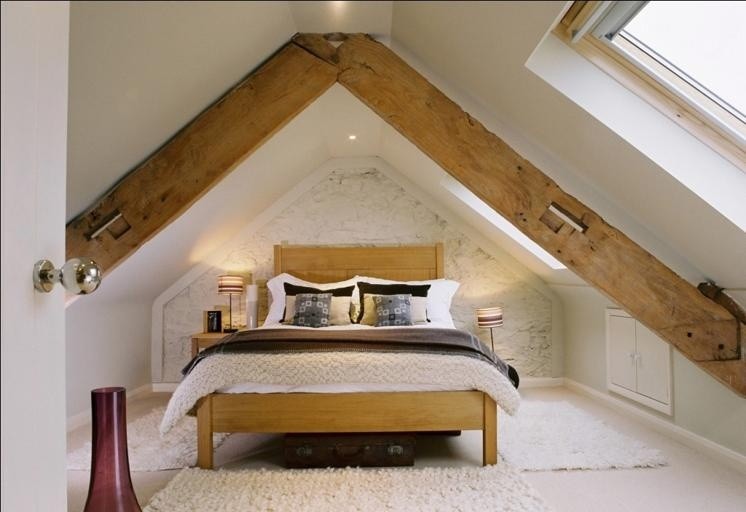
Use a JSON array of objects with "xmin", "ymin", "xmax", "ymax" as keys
[{"xmin": 202, "ymin": 310, "xmax": 221, "ymax": 334}]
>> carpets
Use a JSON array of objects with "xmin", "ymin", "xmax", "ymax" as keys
[
  {"xmin": 496, "ymin": 400, "xmax": 666, "ymax": 472},
  {"xmin": 141, "ymin": 465, "xmax": 556, "ymax": 512},
  {"xmin": 67, "ymin": 405, "xmax": 231, "ymax": 473}
]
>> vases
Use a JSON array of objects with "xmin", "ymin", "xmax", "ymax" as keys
[{"xmin": 82, "ymin": 385, "xmax": 143, "ymax": 512}]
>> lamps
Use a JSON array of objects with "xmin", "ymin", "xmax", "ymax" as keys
[
  {"xmin": 476, "ymin": 306, "xmax": 504, "ymax": 351},
  {"xmin": 215, "ymin": 276, "xmax": 244, "ymax": 331}
]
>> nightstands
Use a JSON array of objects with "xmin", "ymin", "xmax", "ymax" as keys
[{"xmin": 192, "ymin": 334, "xmax": 230, "ymax": 361}]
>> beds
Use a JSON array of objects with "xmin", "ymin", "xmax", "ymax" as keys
[{"xmin": 197, "ymin": 245, "xmax": 496, "ymax": 470}]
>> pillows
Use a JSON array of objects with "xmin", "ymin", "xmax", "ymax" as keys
[{"xmin": 266, "ymin": 273, "xmax": 461, "ymax": 327}]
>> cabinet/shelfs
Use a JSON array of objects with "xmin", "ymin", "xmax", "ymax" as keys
[{"xmin": 605, "ymin": 309, "xmax": 674, "ymax": 417}]
